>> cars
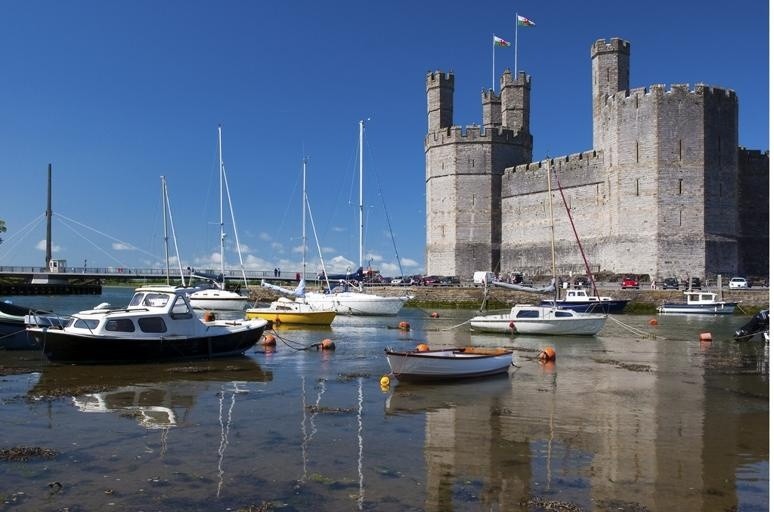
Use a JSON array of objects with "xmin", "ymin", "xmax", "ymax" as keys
[
  {"xmin": 391, "ymin": 275, "xmax": 461, "ymax": 287},
  {"xmin": 662, "ymin": 278, "xmax": 679, "ymax": 290},
  {"xmin": 685, "ymin": 278, "xmax": 702, "ymax": 290},
  {"xmin": 621, "ymin": 275, "xmax": 639, "ymax": 289},
  {"xmin": 729, "ymin": 278, "xmax": 748, "ymax": 290},
  {"xmin": 520, "ymin": 279, "xmax": 533, "ymax": 288},
  {"xmin": 574, "ymin": 277, "xmax": 591, "ymax": 289}
]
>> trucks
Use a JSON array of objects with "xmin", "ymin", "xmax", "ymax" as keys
[{"xmin": 506, "ymin": 272, "xmax": 523, "ymax": 284}]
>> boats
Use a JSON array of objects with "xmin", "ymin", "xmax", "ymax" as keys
[
  {"xmin": 656, "ymin": 292, "xmax": 741, "ymax": 315},
  {"xmin": 384, "ymin": 344, "xmax": 513, "ymax": 385},
  {"xmin": 541, "ymin": 289, "xmax": 630, "ymax": 314}
]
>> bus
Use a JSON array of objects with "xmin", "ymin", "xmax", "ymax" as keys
[{"xmin": 473, "ymin": 271, "xmax": 497, "ymax": 287}]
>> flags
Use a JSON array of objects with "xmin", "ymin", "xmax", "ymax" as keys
[
  {"xmin": 495, "ymin": 36, "xmax": 511, "ymax": 47},
  {"xmin": 518, "ymin": 15, "xmax": 536, "ymax": 27}
]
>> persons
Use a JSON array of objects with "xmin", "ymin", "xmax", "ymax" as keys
[{"xmin": 274, "ymin": 269, "xmax": 280, "ymax": 277}]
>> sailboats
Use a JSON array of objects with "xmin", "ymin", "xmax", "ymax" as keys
[
  {"xmin": 271, "ymin": 120, "xmax": 416, "ymax": 317},
  {"xmin": 469, "ymin": 158, "xmax": 631, "ymax": 336}
]
